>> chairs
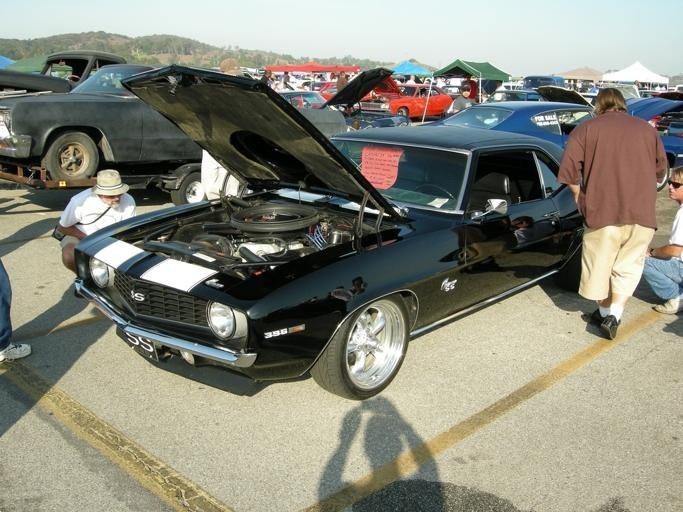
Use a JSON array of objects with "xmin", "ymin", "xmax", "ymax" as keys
[
  {"xmin": 393, "ymin": 161, "xmax": 431, "ymax": 193},
  {"xmin": 467, "ymin": 172, "xmax": 512, "ymax": 212}
]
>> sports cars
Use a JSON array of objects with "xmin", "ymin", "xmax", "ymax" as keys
[{"xmin": 73, "ymin": 64, "xmax": 582, "ymax": 400}]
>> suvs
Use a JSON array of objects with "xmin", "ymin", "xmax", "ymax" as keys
[{"xmin": 0, "ymin": 51, "xmax": 126, "ymax": 102}]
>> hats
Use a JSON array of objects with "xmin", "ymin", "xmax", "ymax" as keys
[
  {"xmin": 92, "ymin": 169, "xmax": 129, "ymax": 196},
  {"xmin": 219, "ymin": 58, "xmax": 241, "ymax": 75}
]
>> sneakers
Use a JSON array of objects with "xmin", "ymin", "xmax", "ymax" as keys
[
  {"xmin": 0, "ymin": 342, "xmax": 31, "ymax": 362},
  {"xmin": 591, "ymin": 308, "xmax": 620, "ymax": 340},
  {"xmin": 654, "ymin": 299, "xmax": 683, "ymax": 314}
]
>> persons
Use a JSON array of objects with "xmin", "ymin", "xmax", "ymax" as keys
[
  {"xmin": 201, "ymin": 58, "xmax": 242, "ymax": 212},
  {"xmin": 641, "ymin": 166, "xmax": 683, "ymax": 315},
  {"xmin": 0, "ymin": 261, "xmax": 32, "ymax": 362},
  {"xmin": 511, "ymin": 216, "xmax": 556, "ymax": 246},
  {"xmin": 261, "ymin": 69, "xmax": 507, "ymax": 115},
  {"xmin": 56, "ymin": 169, "xmax": 136, "ymax": 298},
  {"xmin": 556, "ymin": 88, "xmax": 668, "ymax": 340}
]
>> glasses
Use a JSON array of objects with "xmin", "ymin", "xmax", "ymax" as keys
[{"xmin": 668, "ymin": 178, "xmax": 683, "ymax": 188}]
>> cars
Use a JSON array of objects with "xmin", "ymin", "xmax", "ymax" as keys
[
  {"xmin": 233, "ymin": 64, "xmax": 682, "ymax": 137},
  {"xmin": 0, "ymin": 64, "xmax": 347, "ymax": 176},
  {"xmin": 402, "ymin": 97, "xmax": 682, "ymax": 195},
  {"xmin": 31, "ymin": 68, "xmax": 96, "ymax": 82}
]
